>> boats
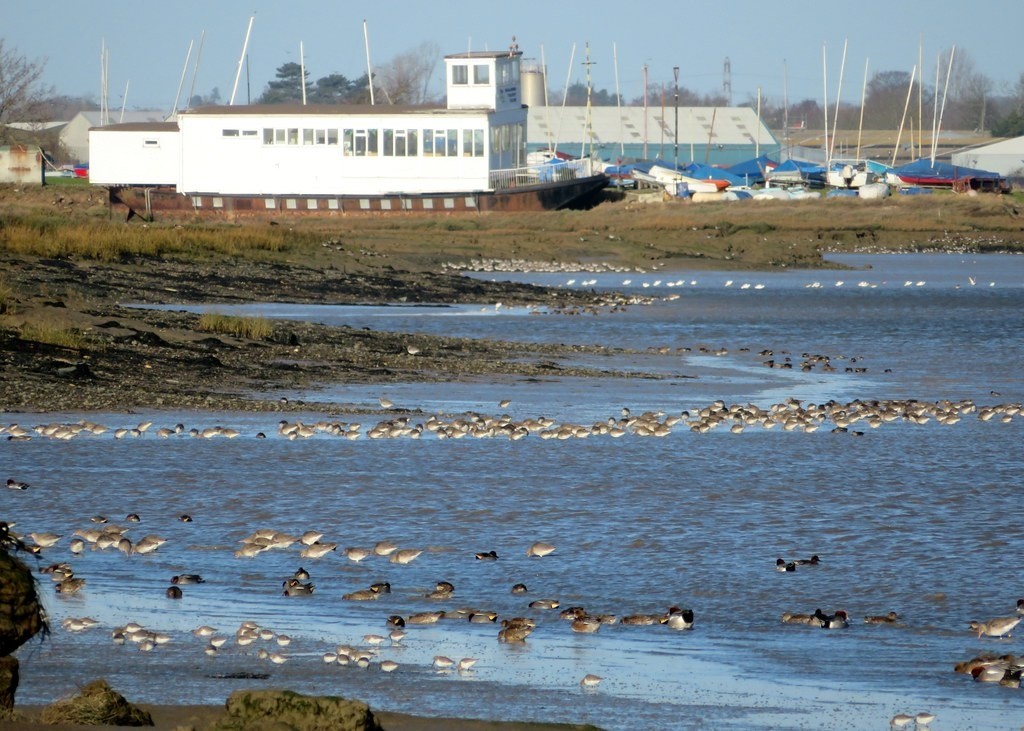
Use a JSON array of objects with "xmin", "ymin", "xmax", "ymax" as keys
[{"xmin": 71, "ymin": 43, "xmax": 1014, "ymax": 219}]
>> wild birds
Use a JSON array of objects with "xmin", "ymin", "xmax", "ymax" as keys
[
  {"xmin": 780, "ymin": 608, "xmax": 850, "ymax": 629},
  {"xmin": 864, "ymin": 611, "xmax": 902, "ymax": 626},
  {"xmin": 955, "ymin": 652, "xmax": 1024, "ymax": 689},
  {"xmin": 4, "ymin": 478, "xmax": 31, "ymax": 491},
  {"xmin": 911, "ymin": 713, "xmax": 938, "ymax": 729},
  {"xmin": 443, "ymin": 223, "xmax": 1024, "ymax": 313},
  {"xmin": 773, "ymin": 555, "xmax": 823, "ymax": 573},
  {"xmin": 968, "ymin": 598, "xmax": 1024, "ymax": 637},
  {"xmin": 890, "ymin": 715, "xmax": 916, "ymax": 729},
  {"xmin": 578, "ymin": 673, "xmax": 603, "ymax": 688},
  {"xmin": 1, "ymin": 344, "xmax": 1024, "ymax": 440},
  {"xmin": 0, "ymin": 513, "xmax": 695, "ymax": 671}
]
